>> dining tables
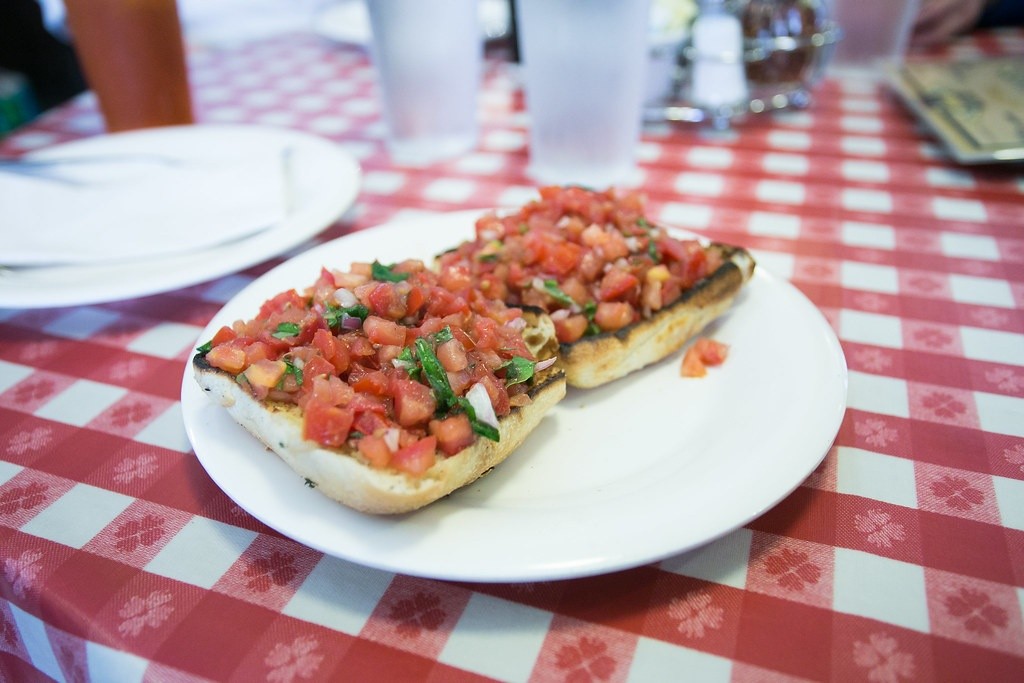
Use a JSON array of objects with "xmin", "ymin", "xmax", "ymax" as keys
[{"xmin": 0, "ymin": 29, "xmax": 1024, "ymax": 682}]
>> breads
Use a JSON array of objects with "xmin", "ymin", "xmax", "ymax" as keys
[{"xmin": 190, "ymin": 189, "xmax": 754, "ymax": 512}]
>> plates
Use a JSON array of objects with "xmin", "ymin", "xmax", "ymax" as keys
[
  {"xmin": 181, "ymin": 209, "xmax": 847, "ymax": 582},
  {"xmin": 0, "ymin": 123, "xmax": 364, "ymax": 308}
]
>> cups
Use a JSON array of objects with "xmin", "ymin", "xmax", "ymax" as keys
[
  {"xmin": 367, "ymin": 0, "xmax": 488, "ymax": 170},
  {"xmin": 62, "ymin": 1, "xmax": 196, "ymax": 133},
  {"xmin": 513, "ymin": 2, "xmax": 641, "ymax": 194}
]
